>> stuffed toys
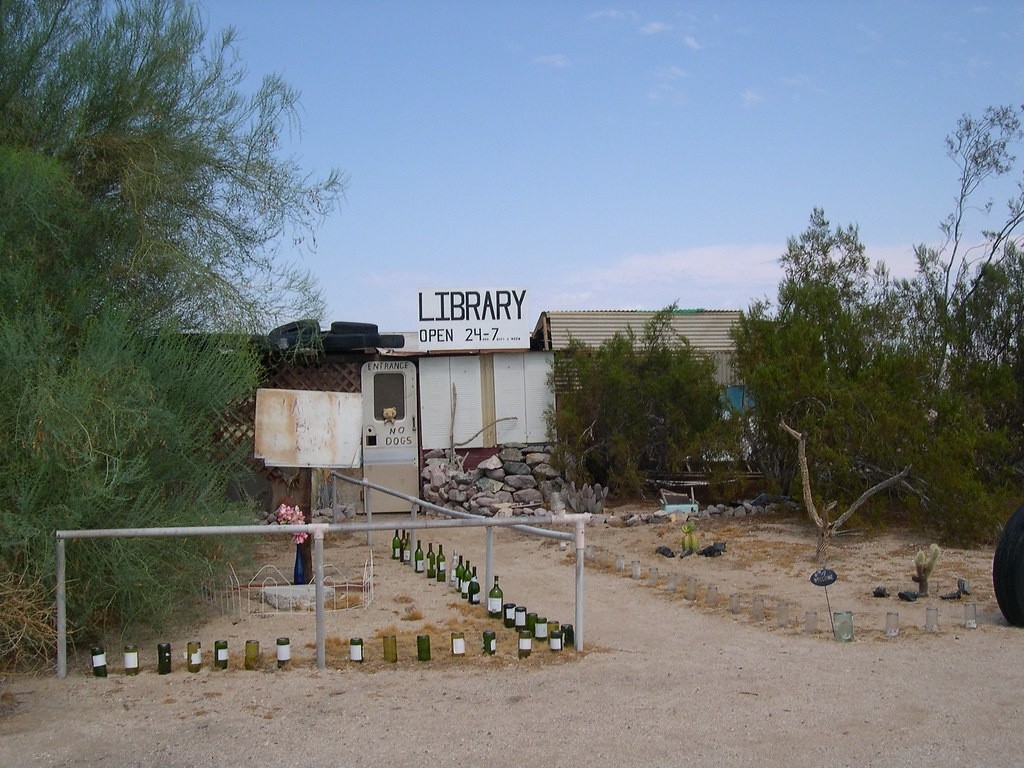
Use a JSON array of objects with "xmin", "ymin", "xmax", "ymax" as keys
[{"xmin": 382, "ymin": 407, "xmax": 396, "ymax": 424}]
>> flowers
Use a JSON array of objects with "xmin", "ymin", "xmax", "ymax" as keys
[{"xmin": 275, "ymin": 504, "xmax": 308, "ymax": 545}]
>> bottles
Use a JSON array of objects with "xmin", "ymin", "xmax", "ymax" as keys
[
  {"xmin": 448, "ymin": 550, "xmax": 480, "ymax": 605},
  {"xmin": 437, "ymin": 544, "xmax": 446, "ymax": 583},
  {"xmin": 244, "ymin": 639, "xmax": 260, "ymax": 671},
  {"xmin": 123, "ymin": 643, "xmax": 139, "ymax": 676},
  {"xmin": 382, "ymin": 635, "xmax": 398, "ymax": 664},
  {"xmin": 392, "ymin": 529, "xmax": 410, "ymax": 565},
  {"xmin": 488, "ymin": 575, "xmax": 504, "ymax": 619},
  {"xmin": 504, "ymin": 603, "xmax": 574, "ymax": 648},
  {"xmin": 348, "ymin": 638, "xmax": 364, "ymax": 663},
  {"xmin": 482, "ymin": 628, "xmax": 566, "ymax": 659},
  {"xmin": 156, "ymin": 642, "xmax": 172, "ymax": 675},
  {"xmin": 214, "ymin": 640, "xmax": 229, "ymax": 671},
  {"xmin": 91, "ymin": 645, "xmax": 108, "ymax": 677},
  {"xmin": 450, "ymin": 632, "xmax": 467, "ymax": 657},
  {"xmin": 276, "ymin": 637, "xmax": 291, "ymax": 670},
  {"xmin": 426, "ymin": 542, "xmax": 435, "ymax": 578},
  {"xmin": 414, "ymin": 540, "xmax": 424, "ymax": 573},
  {"xmin": 417, "ymin": 634, "xmax": 432, "ymax": 662},
  {"xmin": 187, "ymin": 640, "xmax": 203, "ymax": 673}
]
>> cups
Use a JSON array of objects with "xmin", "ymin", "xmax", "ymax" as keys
[
  {"xmin": 685, "ymin": 577, "xmax": 697, "ymax": 599},
  {"xmin": 805, "ymin": 610, "xmax": 818, "ymax": 634},
  {"xmin": 963, "ymin": 603, "xmax": 978, "ymax": 628},
  {"xmin": 777, "ymin": 603, "xmax": 790, "ymax": 626},
  {"xmin": 666, "ymin": 572, "xmax": 678, "ymax": 592},
  {"xmin": 615, "ymin": 554, "xmax": 625, "ymax": 572},
  {"xmin": 752, "ymin": 598, "xmax": 765, "ymax": 622},
  {"xmin": 729, "ymin": 594, "xmax": 741, "ymax": 613},
  {"xmin": 833, "ymin": 611, "xmax": 853, "ymax": 642},
  {"xmin": 531, "ymin": 534, "xmax": 610, "ymax": 569},
  {"xmin": 631, "ymin": 560, "xmax": 641, "ymax": 580},
  {"xmin": 925, "ymin": 607, "xmax": 940, "ymax": 631},
  {"xmin": 648, "ymin": 566, "xmax": 658, "ymax": 586},
  {"xmin": 706, "ymin": 585, "xmax": 719, "ymax": 607},
  {"xmin": 886, "ymin": 612, "xmax": 900, "ymax": 637}
]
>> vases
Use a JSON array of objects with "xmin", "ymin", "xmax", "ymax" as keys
[{"xmin": 294, "ymin": 543, "xmax": 305, "ymax": 585}]
